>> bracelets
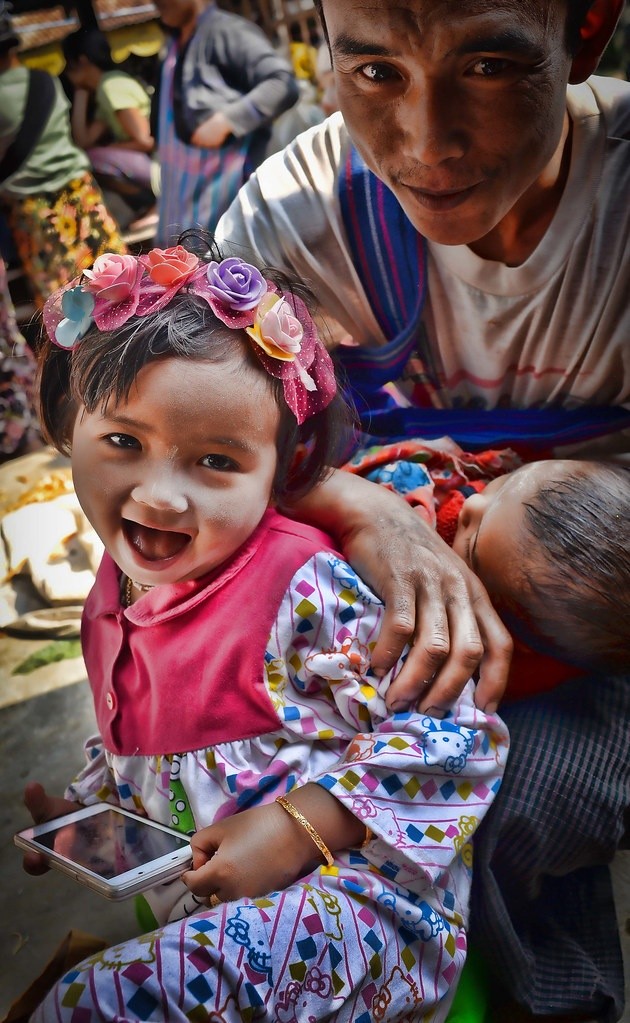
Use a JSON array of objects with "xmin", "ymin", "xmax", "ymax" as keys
[
  {"xmin": 343, "ymin": 823, "xmax": 372, "ymax": 852},
  {"xmin": 275, "ymin": 794, "xmax": 335, "ymax": 868}
]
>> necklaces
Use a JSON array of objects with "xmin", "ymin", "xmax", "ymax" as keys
[
  {"xmin": 121, "ymin": 577, "xmax": 133, "ymax": 609},
  {"xmin": 131, "ymin": 579, "xmax": 155, "ymax": 592}
]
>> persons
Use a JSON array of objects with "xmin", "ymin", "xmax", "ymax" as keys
[
  {"xmin": 60, "ymin": 22, "xmax": 163, "ymax": 232},
  {"xmin": 2, "ymin": 8, "xmax": 129, "ymax": 324},
  {"xmin": 14, "ymin": 244, "xmax": 512, "ymax": 1019},
  {"xmin": 150, "ymin": 0, "xmax": 298, "ymax": 259},
  {"xmin": 213, "ymin": 1, "xmax": 629, "ymax": 1022},
  {"xmin": 336, "ymin": 422, "xmax": 629, "ymax": 702}
]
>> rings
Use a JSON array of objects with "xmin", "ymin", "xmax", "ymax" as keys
[{"xmin": 209, "ymin": 892, "xmax": 223, "ymax": 908}]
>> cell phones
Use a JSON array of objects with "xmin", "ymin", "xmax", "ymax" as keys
[{"xmin": 13, "ymin": 800, "xmax": 193, "ymax": 903}]
